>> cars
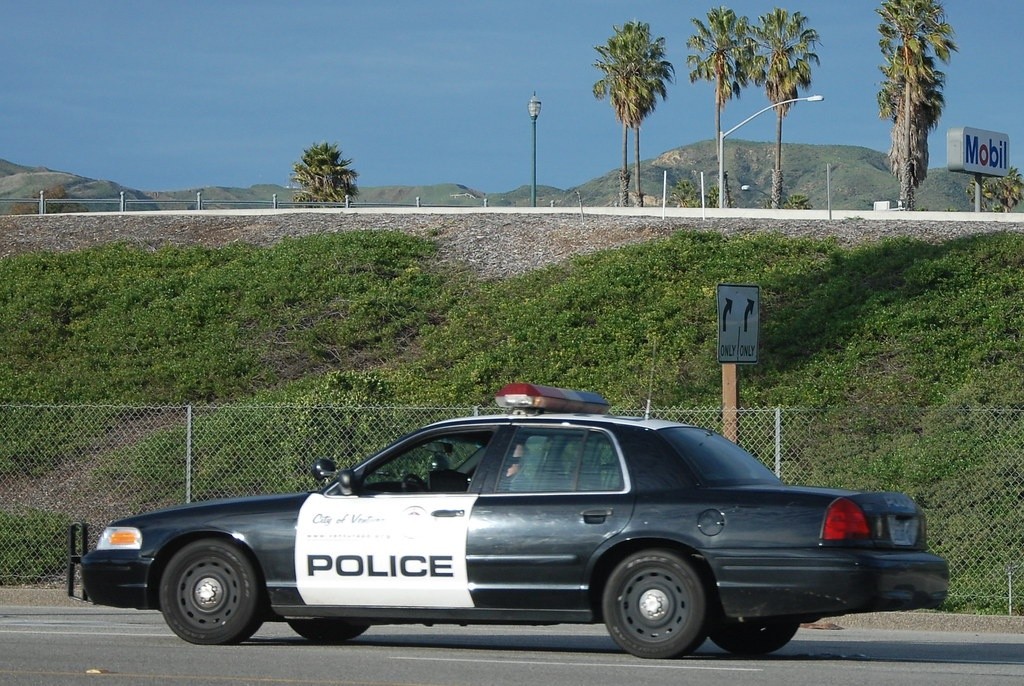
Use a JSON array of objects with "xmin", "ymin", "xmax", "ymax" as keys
[{"xmin": 78, "ymin": 382, "xmax": 951, "ymax": 659}]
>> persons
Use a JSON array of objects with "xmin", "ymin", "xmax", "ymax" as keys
[{"xmin": 506, "ymin": 443, "xmax": 529, "ymax": 493}]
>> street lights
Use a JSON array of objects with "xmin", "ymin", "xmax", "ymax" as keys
[
  {"xmin": 527, "ymin": 96, "xmax": 542, "ymax": 207},
  {"xmin": 740, "ymin": 185, "xmax": 783, "ymax": 209},
  {"xmin": 716, "ymin": 94, "xmax": 825, "ymax": 208}
]
